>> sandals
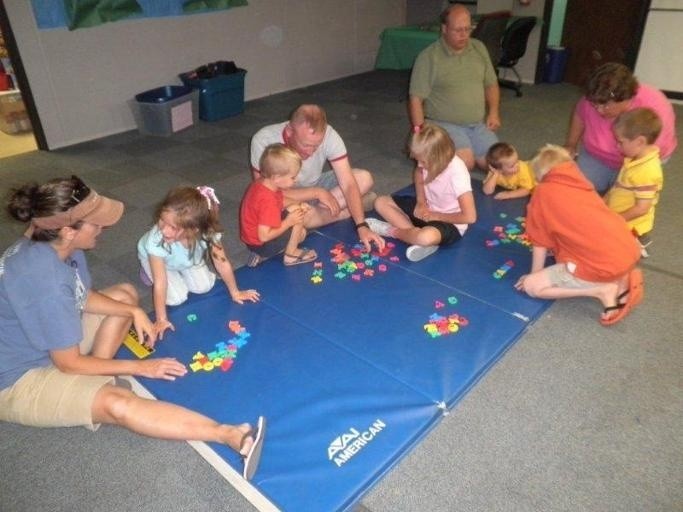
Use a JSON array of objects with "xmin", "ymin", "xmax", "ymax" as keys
[
  {"xmin": 284, "ymin": 250, "xmax": 317, "ymax": 266},
  {"xmin": 248, "ymin": 252, "xmax": 260, "ymax": 267}
]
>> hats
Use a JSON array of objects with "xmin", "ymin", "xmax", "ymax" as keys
[{"xmin": 24, "ymin": 188, "xmax": 124, "ymax": 238}]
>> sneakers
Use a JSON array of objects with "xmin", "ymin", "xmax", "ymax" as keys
[
  {"xmin": 365, "ymin": 217, "xmax": 392, "ymax": 237},
  {"xmin": 406, "ymin": 245, "xmax": 439, "ymax": 261}
]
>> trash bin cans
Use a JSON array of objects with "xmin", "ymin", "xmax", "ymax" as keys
[{"xmin": 543, "ymin": 46, "xmax": 567, "ymax": 83}]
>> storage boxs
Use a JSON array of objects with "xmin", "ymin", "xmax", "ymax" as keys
[{"xmin": 128, "ymin": 61, "xmax": 247, "ymax": 139}]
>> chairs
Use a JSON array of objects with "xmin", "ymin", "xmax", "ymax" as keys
[{"xmin": 470, "ymin": 10, "xmax": 538, "ymax": 98}]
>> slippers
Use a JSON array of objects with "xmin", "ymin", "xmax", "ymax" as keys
[
  {"xmin": 240, "ymin": 416, "xmax": 263, "ymax": 480},
  {"xmin": 601, "ymin": 269, "xmax": 643, "ymax": 325}
]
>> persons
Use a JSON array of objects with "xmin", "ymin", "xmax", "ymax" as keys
[
  {"xmin": 235, "ymin": 141, "xmax": 317, "ymax": 269},
  {"xmin": 406, "ymin": 3, "xmax": 503, "ymax": 176},
  {"xmin": 511, "ymin": 140, "xmax": 644, "ymax": 325},
  {"xmin": 559, "ymin": 61, "xmax": 678, "ymax": 194},
  {"xmin": 136, "ymin": 183, "xmax": 261, "ymax": 340},
  {"xmin": 599, "ymin": 106, "xmax": 665, "ymax": 260},
  {"xmin": 363, "ymin": 120, "xmax": 477, "ymax": 263},
  {"xmin": 1, "ymin": 174, "xmax": 257, "ymax": 472},
  {"xmin": 250, "ymin": 102, "xmax": 379, "ymax": 230},
  {"xmin": 480, "ymin": 142, "xmax": 539, "ymax": 201}
]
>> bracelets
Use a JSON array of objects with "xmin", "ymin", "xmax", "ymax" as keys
[{"xmin": 354, "ymin": 221, "xmax": 370, "ymax": 233}]
